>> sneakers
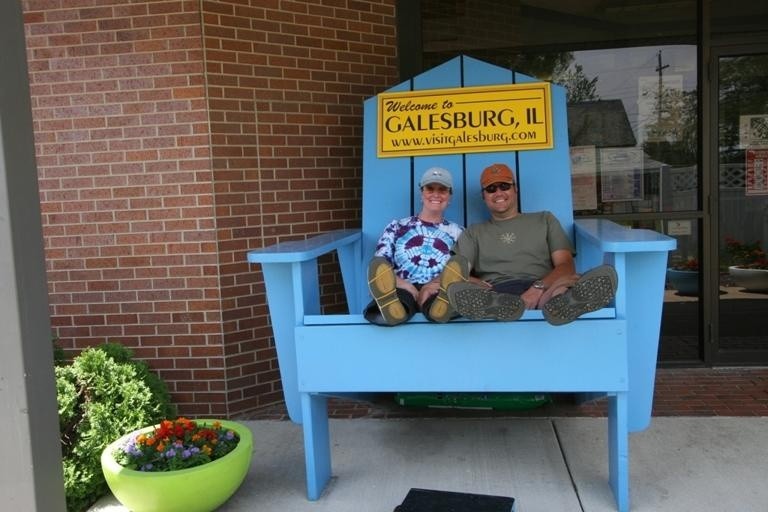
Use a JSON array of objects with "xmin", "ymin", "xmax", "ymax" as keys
[
  {"xmin": 446, "ymin": 280, "xmax": 524, "ymax": 320},
  {"xmin": 428, "ymin": 254, "xmax": 469, "ymax": 322},
  {"xmin": 366, "ymin": 256, "xmax": 410, "ymax": 326},
  {"xmin": 542, "ymin": 265, "xmax": 618, "ymax": 326}
]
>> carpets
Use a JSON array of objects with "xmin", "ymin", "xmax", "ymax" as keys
[{"xmin": 393, "ymin": 487, "xmax": 515, "ymax": 512}]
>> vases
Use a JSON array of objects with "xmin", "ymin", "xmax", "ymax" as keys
[
  {"xmin": 101, "ymin": 420, "xmax": 252, "ymax": 512},
  {"xmin": 728, "ymin": 265, "xmax": 768, "ymax": 291},
  {"xmin": 668, "ymin": 267, "xmax": 698, "ymax": 295}
]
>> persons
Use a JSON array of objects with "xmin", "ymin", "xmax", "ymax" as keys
[
  {"xmin": 446, "ymin": 163, "xmax": 618, "ymax": 327},
  {"xmin": 362, "ymin": 165, "xmax": 465, "ymax": 327}
]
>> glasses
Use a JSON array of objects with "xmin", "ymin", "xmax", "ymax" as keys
[{"xmin": 483, "ymin": 183, "xmax": 512, "ymax": 193}]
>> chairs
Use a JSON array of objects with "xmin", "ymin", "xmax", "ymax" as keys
[{"xmin": 247, "ymin": 55, "xmax": 678, "ymax": 512}]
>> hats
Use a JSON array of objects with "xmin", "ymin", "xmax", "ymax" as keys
[
  {"xmin": 480, "ymin": 163, "xmax": 514, "ymax": 189},
  {"xmin": 421, "ymin": 167, "xmax": 453, "ymax": 188}
]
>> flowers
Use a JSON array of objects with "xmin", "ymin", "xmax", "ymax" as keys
[
  {"xmin": 673, "ymin": 254, "xmax": 698, "ymax": 271},
  {"xmin": 112, "ymin": 417, "xmax": 239, "ymax": 473},
  {"xmin": 723, "ymin": 233, "xmax": 768, "ymax": 270}
]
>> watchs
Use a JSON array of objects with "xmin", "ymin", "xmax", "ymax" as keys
[{"xmin": 533, "ymin": 280, "xmax": 546, "ymax": 290}]
